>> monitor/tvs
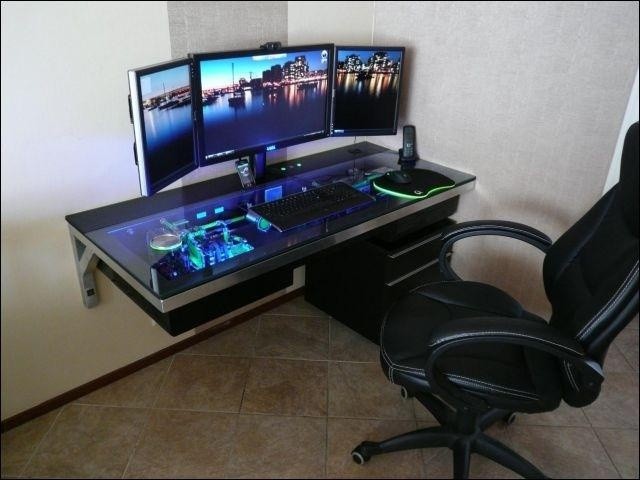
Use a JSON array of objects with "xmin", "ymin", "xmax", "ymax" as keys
[
  {"xmin": 329, "ymin": 46, "xmax": 405, "ymax": 138},
  {"xmin": 127, "ymin": 57, "xmax": 200, "ymax": 199},
  {"xmin": 186, "ymin": 42, "xmax": 334, "ymax": 169}
]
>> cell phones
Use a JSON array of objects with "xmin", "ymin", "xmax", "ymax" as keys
[{"xmin": 235, "ymin": 159, "xmax": 257, "ymax": 188}]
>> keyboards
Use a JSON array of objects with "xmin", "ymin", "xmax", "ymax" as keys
[{"xmin": 248, "ymin": 181, "xmax": 375, "ymax": 234}]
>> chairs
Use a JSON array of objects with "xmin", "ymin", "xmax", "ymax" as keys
[{"xmin": 350, "ymin": 121, "xmax": 640, "ymax": 480}]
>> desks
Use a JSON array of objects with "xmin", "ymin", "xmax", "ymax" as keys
[{"xmin": 65, "ymin": 140, "xmax": 477, "ymax": 347}]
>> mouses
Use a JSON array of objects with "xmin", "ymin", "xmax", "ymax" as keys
[{"xmin": 387, "ymin": 170, "xmax": 413, "ymax": 185}]
